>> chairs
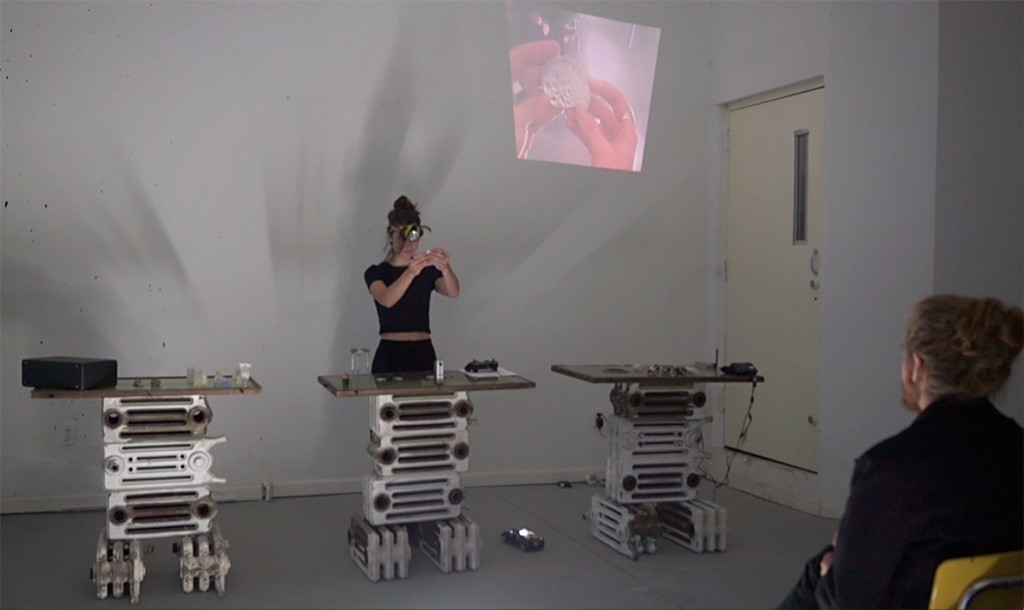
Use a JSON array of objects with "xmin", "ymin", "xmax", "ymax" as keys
[{"xmin": 927, "ymin": 551, "xmax": 1024, "ymax": 610}]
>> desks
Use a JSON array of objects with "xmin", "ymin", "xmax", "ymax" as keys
[
  {"xmin": 549, "ymin": 361, "xmax": 765, "ymax": 562},
  {"xmin": 30, "ymin": 375, "xmax": 263, "ymax": 604},
  {"xmin": 317, "ymin": 367, "xmax": 537, "ymax": 581}
]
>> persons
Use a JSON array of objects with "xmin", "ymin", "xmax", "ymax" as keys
[
  {"xmin": 774, "ymin": 293, "xmax": 1024, "ymax": 610},
  {"xmin": 362, "ymin": 195, "xmax": 460, "ymax": 375},
  {"xmin": 508, "ymin": 39, "xmax": 638, "ymax": 172}
]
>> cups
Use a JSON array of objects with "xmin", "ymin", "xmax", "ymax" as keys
[{"xmin": 239, "ymin": 362, "xmax": 253, "ymax": 381}]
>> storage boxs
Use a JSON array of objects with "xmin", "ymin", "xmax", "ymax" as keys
[{"xmin": 22, "ymin": 355, "xmax": 118, "ymax": 392}]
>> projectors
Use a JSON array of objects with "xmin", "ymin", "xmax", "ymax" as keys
[{"xmin": 21, "ymin": 354, "xmax": 117, "ymax": 390}]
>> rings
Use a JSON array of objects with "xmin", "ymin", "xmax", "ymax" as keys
[
  {"xmin": 410, "ymin": 256, "xmax": 414, "ymax": 261},
  {"xmin": 617, "ymin": 107, "xmax": 636, "ymax": 122},
  {"xmin": 512, "ymin": 79, "xmax": 526, "ymax": 102},
  {"xmin": 442, "ymin": 255, "xmax": 446, "ymax": 259}
]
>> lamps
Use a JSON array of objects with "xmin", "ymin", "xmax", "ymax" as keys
[{"xmin": 400, "ymin": 222, "xmax": 424, "ymax": 242}]
